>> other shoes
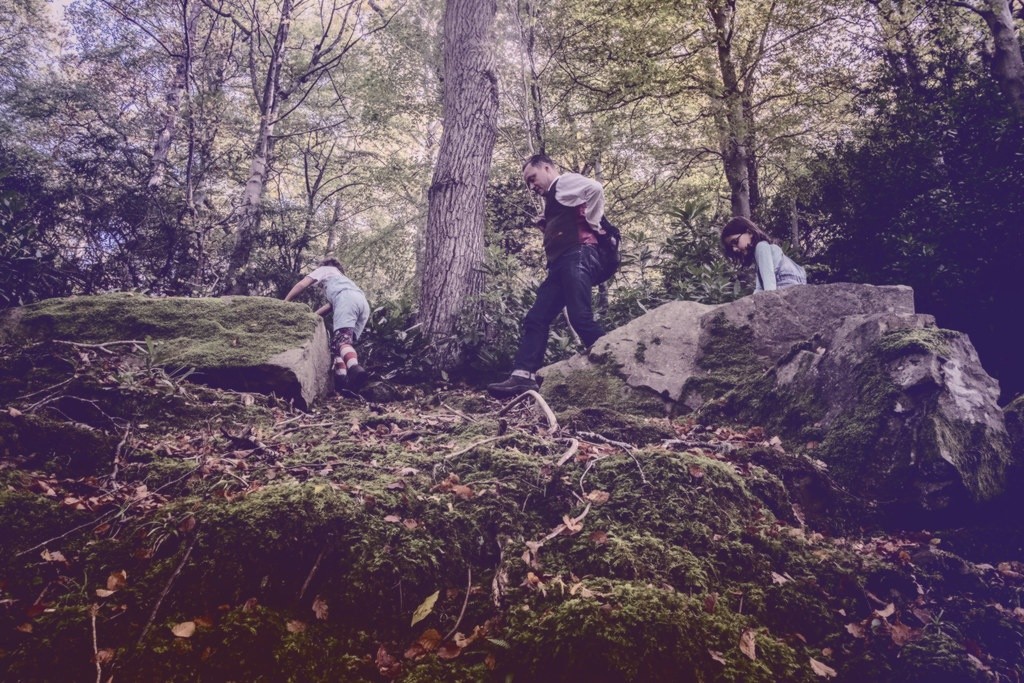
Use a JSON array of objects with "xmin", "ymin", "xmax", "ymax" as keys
[{"xmin": 335, "ymin": 366, "xmax": 367, "ymax": 396}]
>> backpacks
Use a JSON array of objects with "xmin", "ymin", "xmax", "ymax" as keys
[{"xmin": 593, "ymin": 208, "xmax": 622, "ymax": 285}]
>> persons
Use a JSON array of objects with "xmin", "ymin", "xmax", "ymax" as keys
[
  {"xmin": 488, "ymin": 154, "xmax": 606, "ymax": 399},
  {"xmin": 721, "ymin": 216, "xmax": 806, "ymax": 294},
  {"xmin": 283, "ymin": 256, "xmax": 370, "ymax": 395}
]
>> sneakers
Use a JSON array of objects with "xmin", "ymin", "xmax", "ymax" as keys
[{"xmin": 487, "ymin": 375, "xmax": 540, "ymax": 398}]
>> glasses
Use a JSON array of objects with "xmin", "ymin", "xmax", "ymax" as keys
[{"xmin": 727, "ymin": 233, "xmax": 744, "ymax": 252}]
